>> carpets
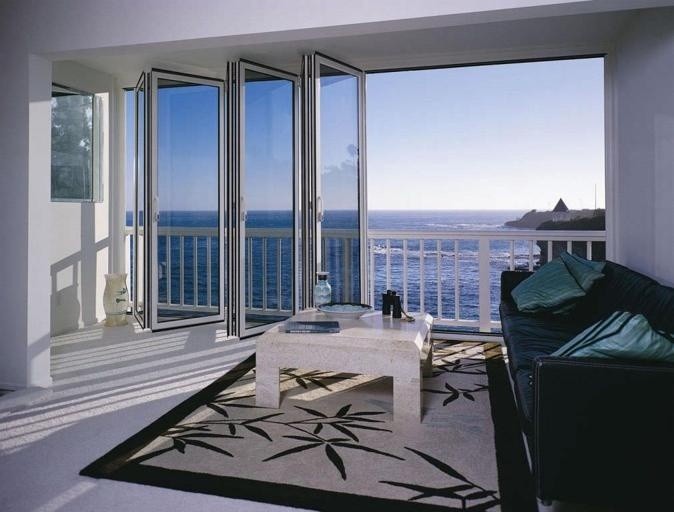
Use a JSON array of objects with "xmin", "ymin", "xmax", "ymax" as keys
[{"xmin": 76, "ymin": 338, "xmax": 541, "ymax": 512}]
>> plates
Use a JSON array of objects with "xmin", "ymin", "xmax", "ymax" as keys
[{"xmin": 317, "ymin": 302, "xmax": 374, "ymax": 320}]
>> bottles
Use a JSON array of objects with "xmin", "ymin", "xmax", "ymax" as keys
[{"xmin": 314, "ymin": 272, "xmax": 332, "ymax": 314}]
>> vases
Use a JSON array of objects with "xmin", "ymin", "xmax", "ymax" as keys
[
  {"xmin": 99, "ymin": 272, "xmax": 132, "ymax": 328},
  {"xmin": 314, "ymin": 271, "xmax": 331, "ymax": 306}
]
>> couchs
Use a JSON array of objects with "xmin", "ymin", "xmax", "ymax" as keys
[{"xmin": 498, "ymin": 256, "xmax": 674, "ymax": 509}]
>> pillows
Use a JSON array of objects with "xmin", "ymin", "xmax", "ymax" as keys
[
  {"xmin": 510, "ymin": 252, "xmax": 605, "ymax": 311},
  {"xmin": 552, "ymin": 312, "xmax": 674, "ymax": 357}
]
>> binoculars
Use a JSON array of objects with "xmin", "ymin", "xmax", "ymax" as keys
[{"xmin": 381, "ymin": 290, "xmax": 401, "ymax": 318}]
